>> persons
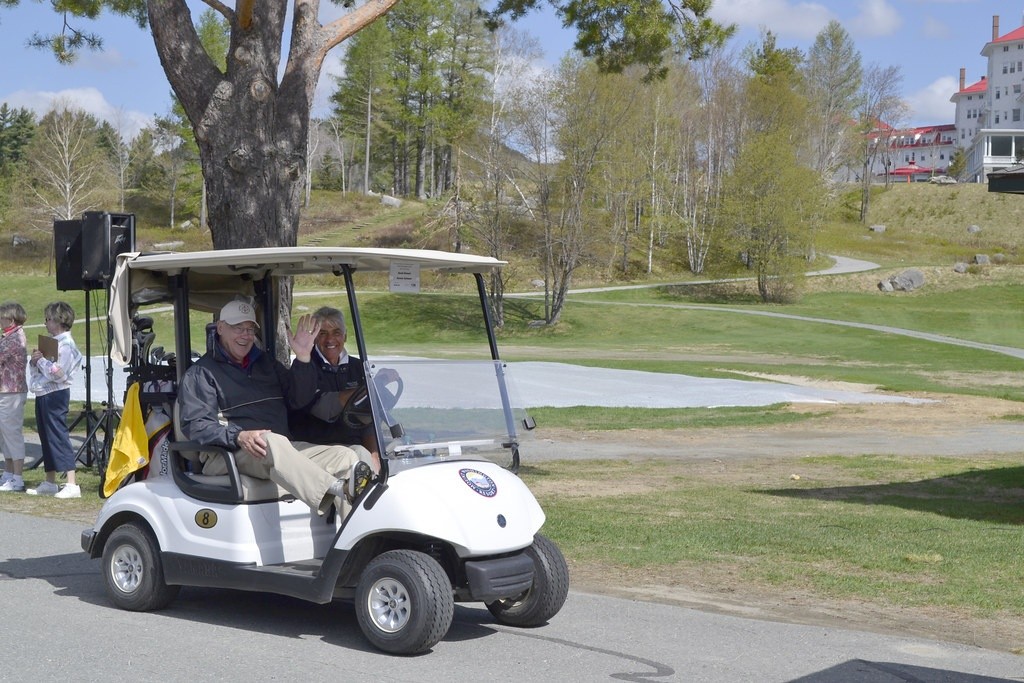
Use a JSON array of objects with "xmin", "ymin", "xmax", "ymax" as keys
[
  {"xmin": 26, "ymin": 301, "xmax": 81, "ymax": 499},
  {"xmin": 287, "ymin": 307, "xmax": 382, "ymax": 477},
  {"xmin": 0, "ymin": 302, "xmax": 29, "ymax": 491},
  {"xmin": 178, "ymin": 300, "xmax": 376, "ymax": 521}
]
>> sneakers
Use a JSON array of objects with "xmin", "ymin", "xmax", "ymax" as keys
[
  {"xmin": 26, "ymin": 482, "xmax": 59, "ymax": 496},
  {"xmin": 0, "ymin": 476, "xmax": 26, "ymax": 491},
  {"xmin": 347, "ymin": 460, "xmax": 371, "ymax": 506},
  {"xmin": 54, "ymin": 483, "xmax": 81, "ymax": 499}
]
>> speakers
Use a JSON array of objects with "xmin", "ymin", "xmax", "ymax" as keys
[
  {"xmin": 82, "ymin": 210, "xmax": 136, "ymax": 281},
  {"xmin": 54, "ymin": 219, "xmax": 108, "ymax": 292}
]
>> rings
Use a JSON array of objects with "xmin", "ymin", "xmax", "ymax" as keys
[{"xmin": 307, "ymin": 329, "xmax": 312, "ymax": 333}]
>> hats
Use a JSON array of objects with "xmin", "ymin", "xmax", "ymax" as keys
[{"xmin": 219, "ymin": 299, "xmax": 260, "ymax": 329}]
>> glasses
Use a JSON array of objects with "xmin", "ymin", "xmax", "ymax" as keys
[
  {"xmin": 44, "ymin": 317, "xmax": 51, "ymax": 322},
  {"xmin": 223, "ymin": 321, "xmax": 258, "ymax": 335}
]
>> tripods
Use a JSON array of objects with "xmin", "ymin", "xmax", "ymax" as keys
[{"xmin": 29, "ymin": 282, "xmax": 122, "ymax": 480}]
